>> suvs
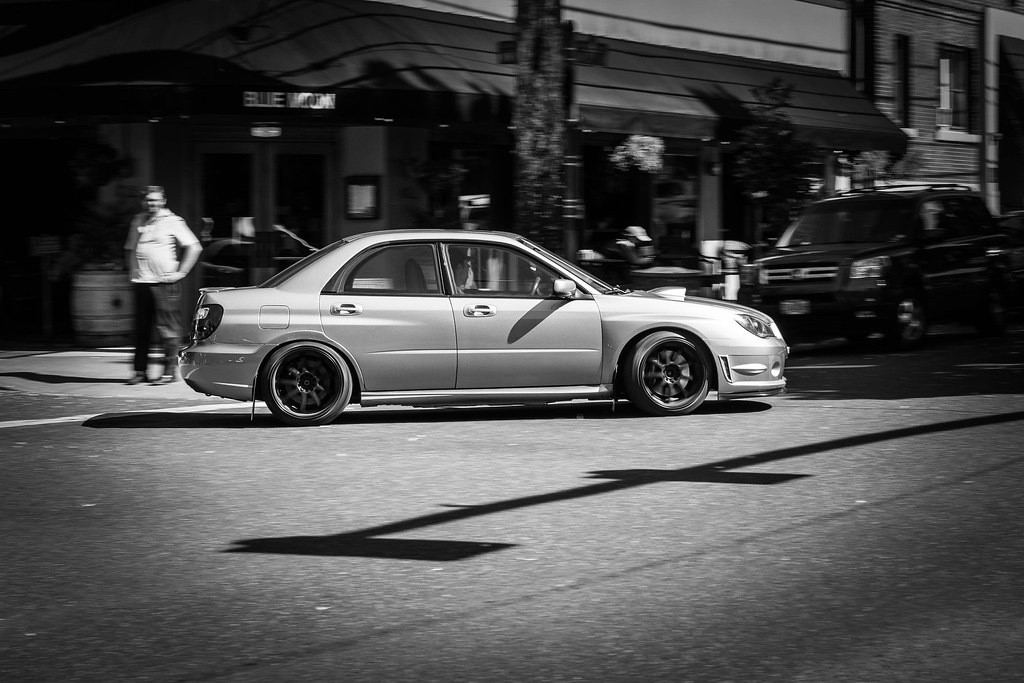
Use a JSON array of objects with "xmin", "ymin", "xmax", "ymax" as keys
[{"xmin": 735, "ymin": 184, "xmax": 998, "ymax": 346}]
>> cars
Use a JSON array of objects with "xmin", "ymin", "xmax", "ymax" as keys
[
  {"xmin": 914, "ymin": 207, "xmax": 1022, "ymax": 345},
  {"xmin": 180, "ymin": 227, "xmax": 791, "ymax": 428}
]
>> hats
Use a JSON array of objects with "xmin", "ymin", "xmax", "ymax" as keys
[{"xmin": 626, "ymin": 226, "xmax": 652, "ymax": 241}]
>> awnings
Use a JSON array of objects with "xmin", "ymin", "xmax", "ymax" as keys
[{"xmin": 194, "ymin": 0, "xmax": 911, "ymax": 155}]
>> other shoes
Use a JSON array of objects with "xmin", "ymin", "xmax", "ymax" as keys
[
  {"xmin": 128, "ymin": 374, "xmax": 147, "ymax": 384},
  {"xmin": 153, "ymin": 374, "xmax": 176, "ymax": 385}
]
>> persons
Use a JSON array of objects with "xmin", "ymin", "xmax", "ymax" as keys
[
  {"xmin": 616, "ymin": 225, "xmax": 658, "ymax": 292},
  {"xmin": 126, "ymin": 184, "xmax": 203, "ymax": 385},
  {"xmin": 449, "ymin": 246, "xmax": 473, "ymax": 295}
]
